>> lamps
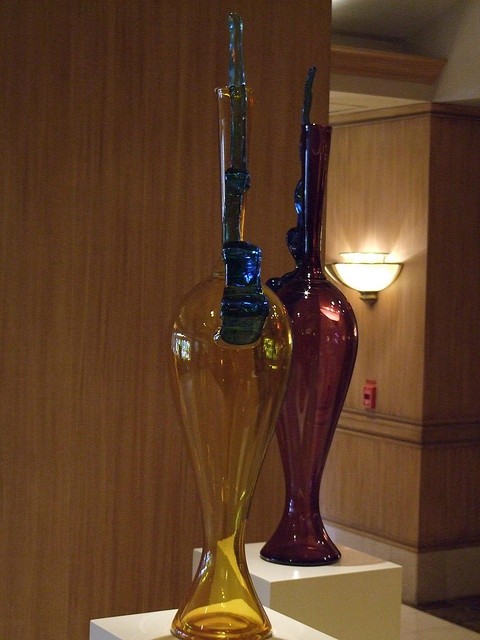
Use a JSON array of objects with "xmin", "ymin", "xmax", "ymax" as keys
[{"xmin": 323, "ymin": 245, "xmax": 400, "ymax": 307}]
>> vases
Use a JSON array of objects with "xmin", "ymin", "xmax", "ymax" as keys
[
  {"xmin": 163, "ymin": 9, "xmax": 294, "ymax": 640},
  {"xmin": 253, "ymin": 58, "xmax": 362, "ymax": 565}
]
